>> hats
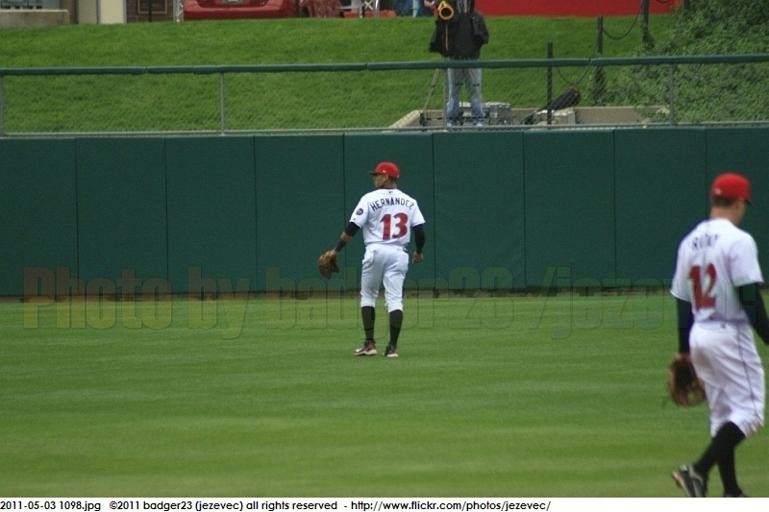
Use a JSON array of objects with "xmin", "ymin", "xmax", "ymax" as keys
[
  {"xmin": 369, "ymin": 161, "xmax": 400, "ymax": 179},
  {"xmin": 709, "ymin": 173, "xmax": 754, "ymax": 205}
]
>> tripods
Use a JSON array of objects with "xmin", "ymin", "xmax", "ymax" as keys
[{"xmin": 419, "ymin": 22, "xmax": 464, "ymax": 132}]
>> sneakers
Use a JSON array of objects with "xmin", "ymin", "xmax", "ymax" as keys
[
  {"xmin": 382, "ymin": 344, "xmax": 399, "ymax": 358},
  {"xmin": 670, "ymin": 464, "xmax": 707, "ymax": 497},
  {"xmin": 353, "ymin": 341, "xmax": 378, "ymax": 356}
]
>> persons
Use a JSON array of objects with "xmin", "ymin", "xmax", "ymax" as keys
[
  {"xmin": 668, "ymin": 172, "xmax": 769, "ymax": 498},
  {"xmin": 316, "ymin": 163, "xmax": 427, "ymax": 358},
  {"xmin": 428, "ymin": 0, "xmax": 490, "ymax": 128}
]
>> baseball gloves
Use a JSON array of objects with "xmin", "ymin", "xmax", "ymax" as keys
[
  {"xmin": 318, "ymin": 251, "xmax": 340, "ymax": 280},
  {"xmin": 667, "ymin": 354, "xmax": 708, "ymax": 407}
]
